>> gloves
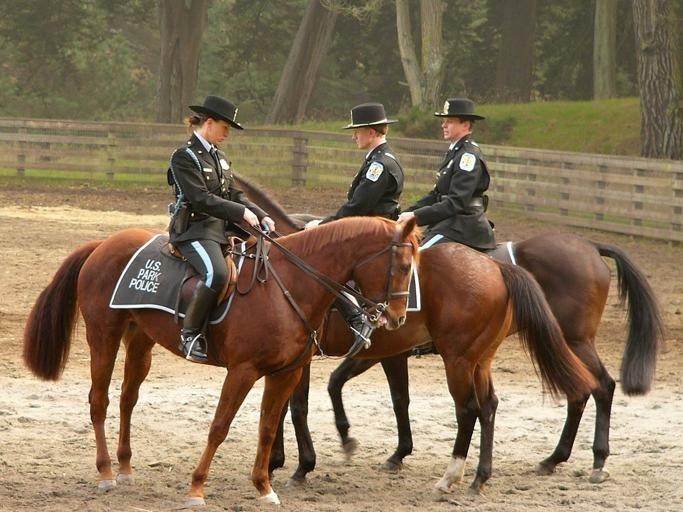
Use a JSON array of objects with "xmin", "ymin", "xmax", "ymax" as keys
[
  {"xmin": 398, "ymin": 211, "xmax": 415, "ymax": 226},
  {"xmin": 305, "ymin": 220, "xmax": 322, "ymax": 229},
  {"xmin": 243, "ymin": 208, "xmax": 259, "ymax": 227},
  {"xmin": 262, "ymin": 215, "xmax": 275, "ymax": 232}
]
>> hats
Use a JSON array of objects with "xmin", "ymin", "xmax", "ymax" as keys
[
  {"xmin": 188, "ymin": 94, "xmax": 244, "ymax": 132},
  {"xmin": 342, "ymin": 102, "xmax": 399, "ymax": 129},
  {"xmin": 434, "ymin": 98, "xmax": 484, "ymax": 120}
]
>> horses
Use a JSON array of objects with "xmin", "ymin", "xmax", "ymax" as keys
[
  {"xmin": 228, "ymin": 167, "xmax": 602, "ymax": 494},
  {"xmin": 22, "ymin": 215, "xmax": 430, "ymax": 509},
  {"xmin": 286, "ymin": 213, "xmax": 667, "ymax": 484}
]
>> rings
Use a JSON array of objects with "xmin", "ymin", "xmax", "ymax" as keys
[{"xmin": 262, "ymin": 224, "xmax": 269, "ymax": 230}]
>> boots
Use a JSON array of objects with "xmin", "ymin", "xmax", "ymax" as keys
[
  {"xmin": 180, "ymin": 280, "xmax": 216, "ymax": 359},
  {"xmin": 335, "ymin": 281, "xmax": 375, "ymax": 348}
]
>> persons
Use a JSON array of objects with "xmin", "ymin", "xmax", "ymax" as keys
[
  {"xmin": 396, "ymin": 96, "xmax": 496, "ymax": 252},
  {"xmin": 302, "ymin": 100, "xmax": 405, "ymax": 359},
  {"xmin": 166, "ymin": 94, "xmax": 276, "ymax": 359}
]
